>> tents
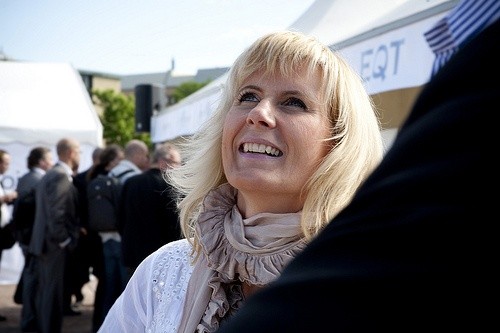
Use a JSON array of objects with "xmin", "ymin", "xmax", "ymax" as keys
[
  {"xmin": 0, "ymin": 58, "xmax": 106, "ymax": 287},
  {"xmin": 151, "ymin": 0, "xmax": 500, "ymax": 145}
]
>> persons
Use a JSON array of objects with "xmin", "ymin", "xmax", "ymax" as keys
[
  {"xmin": 114, "ymin": 140, "xmax": 187, "ymax": 276},
  {"xmin": 29, "ymin": 136, "xmax": 156, "ymax": 333},
  {"xmin": 213, "ymin": 0, "xmax": 500, "ymax": 333},
  {"xmin": 0, "ymin": 149, "xmax": 19, "ymax": 323},
  {"xmin": 96, "ymin": 30, "xmax": 387, "ymax": 333},
  {"xmin": 12, "ymin": 146, "xmax": 55, "ymax": 333}
]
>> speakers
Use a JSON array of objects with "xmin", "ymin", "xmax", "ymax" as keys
[{"xmin": 135, "ymin": 83, "xmax": 165, "ymax": 131}]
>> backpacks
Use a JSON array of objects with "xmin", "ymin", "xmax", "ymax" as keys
[{"xmin": 87, "ymin": 169, "xmax": 135, "ymax": 232}]
